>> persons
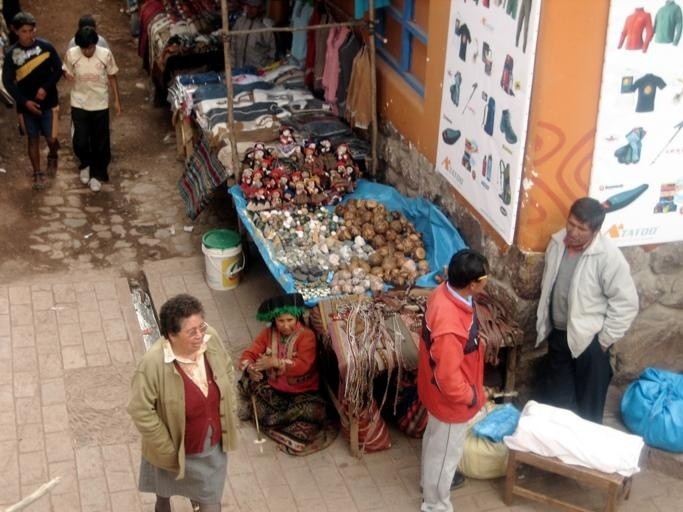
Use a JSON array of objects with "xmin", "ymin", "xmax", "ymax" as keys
[
  {"xmin": 236, "ymin": 121, "xmax": 358, "ymax": 210},
  {"xmin": 61, "ymin": 25, "xmax": 122, "ymax": 193},
  {"xmin": 534, "ymin": 199, "xmax": 641, "ymax": 424},
  {"xmin": 2, "ymin": 14, "xmax": 64, "ymax": 188},
  {"xmin": 237, "ymin": 292, "xmax": 340, "ymax": 455},
  {"xmin": 67, "ymin": 14, "xmax": 109, "ymax": 51},
  {"xmin": 230, "ymin": 2, "xmax": 277, "ymax": 68},
  {"xmin": 415, "ymin": 248, "xmax": 490, "ymax": 512},
  {"xmin": 128, "ymin": 294, "xmax": 234, "ymax": 512}
]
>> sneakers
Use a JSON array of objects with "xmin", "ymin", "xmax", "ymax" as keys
[
  {"xmin": 237, "ymin": 401, "xmax": 252, "ymax": 421},
  {"xmin": 79, "ymin": 166, "xmax": 90, "ymax": 184},
  {"xmin": 87, "ymin": 177, "xmax": 102, "ymax": 192}
]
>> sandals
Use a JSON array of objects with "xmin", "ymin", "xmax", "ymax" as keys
[
  {"xmin": 31, "ymin": 172, "xmax": 45, "ymax": 190},
  {"xmin": 46, "ymin": 155, "xmax": 58, "ymax": 177}
]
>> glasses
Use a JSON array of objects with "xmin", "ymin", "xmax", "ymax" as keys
[
  {"xmin": 478, "ymin": 271, "xmax": 493, "ymax": 281},
  {"xmin": 185, "ymin": 321, "xmax": 208, "ymax": 340}
]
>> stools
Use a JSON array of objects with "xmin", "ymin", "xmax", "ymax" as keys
[{"xmin": 503, "ymin": 399, "xmax": 645, "ymax": 512}]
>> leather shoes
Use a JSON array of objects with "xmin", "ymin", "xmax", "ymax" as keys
[{"xmin": 420, "ymin": 471, "xmax": 468, "ymax": 494}]
